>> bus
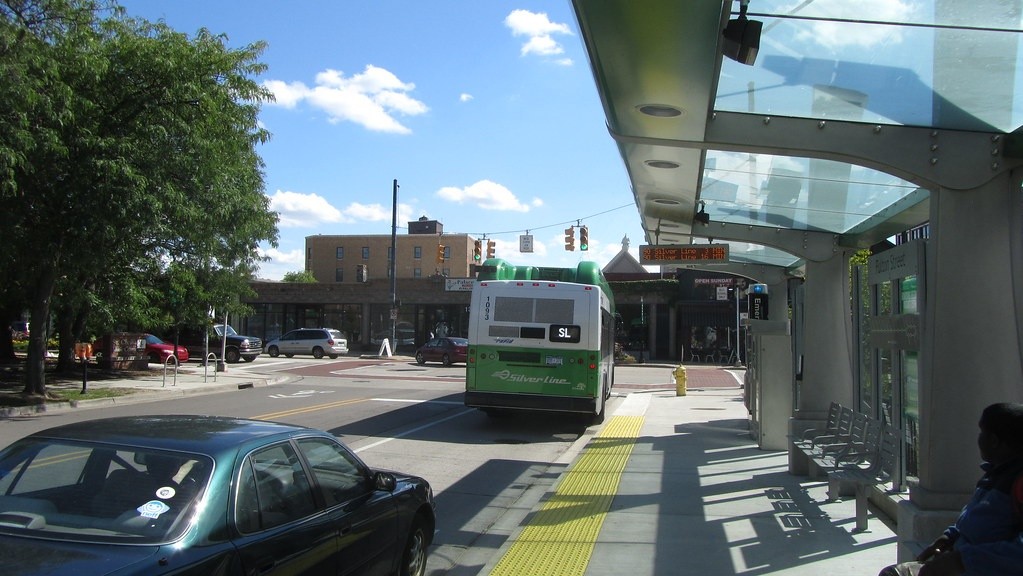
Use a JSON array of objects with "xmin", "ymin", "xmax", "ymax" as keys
[{"xmin": 463, "ymin": 258, "xmax": 626, "ymax": 424}]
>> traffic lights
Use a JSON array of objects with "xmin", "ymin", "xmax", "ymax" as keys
[
  {"xmin": 580, "ymin": 227, "xmax": 588, "ymax": 251},
  {"xmin": 486, "ymin": 240, "xmax": 495, "ymax": 259},
  {"xmin": 437, "ymin": 244, "xmax": 445, "ymax": 263},
  {"xmin": 474, "ymin": 240, "xmax": 482, "ymax": 262},
  {"xmin": 565, "ymin": 225, "xmax": 575, "ymax": 251}
]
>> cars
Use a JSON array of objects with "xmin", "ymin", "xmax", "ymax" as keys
[
  {"xmin": 415, "ymin": 336, "xmax": 468, "ymax": 367},
  {"xmin": 165, "ymin": 323, "xmax": 263, "ymax": 364},
  {"xmin": 92, "ymin": 333, "xmax": 189, "ymax": 364},
  {"xmin": 0, "ymin": 415, "xmax": 436, "ymax": 576},
  {"xmin": 387, "ymin": 319, "xmax": 415, "ymax": 334}
]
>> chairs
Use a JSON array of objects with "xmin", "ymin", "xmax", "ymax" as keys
[
  {"xmin": 787, "ymin": 401, "xmax": 902, "ymax": 533},
  {"xmin": 689, "ymin": 348, "xmax": 729, "ymax": 365},
  {"xmin": 88, "ymin": 468, "xmax": 138, "ymax": 519}
]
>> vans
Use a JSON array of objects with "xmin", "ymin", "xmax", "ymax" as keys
[
  {"xmin": 265, "ymin": 328, "xmax": 349, "ymax": 359},
  {"xmin": 11, "ymin": 321, "xmax": 31, "ymax": 341}
]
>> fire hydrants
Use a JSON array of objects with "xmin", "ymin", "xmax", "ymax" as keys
[{"xmin": 672, "ymin": 364, "xmax": 687, "ymax": 396}]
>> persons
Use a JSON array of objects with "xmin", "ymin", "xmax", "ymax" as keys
[{"xmin": 880, "ymin": 402, "xmax": 1023, "ymax": 576}]
